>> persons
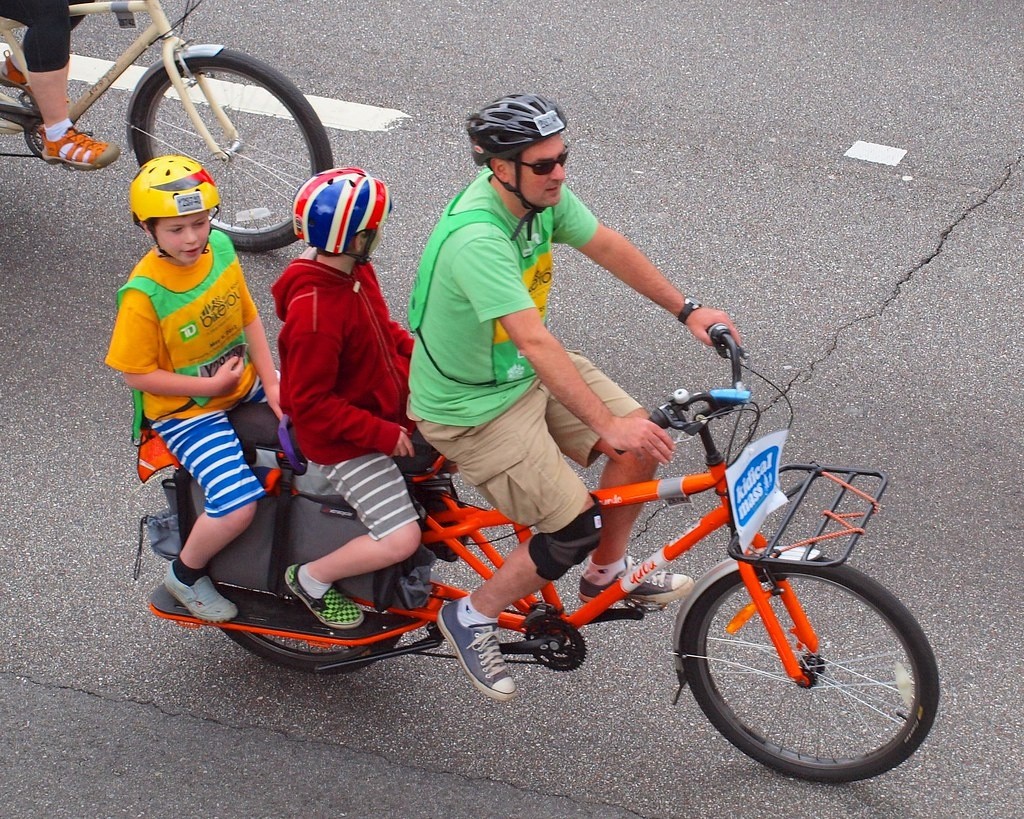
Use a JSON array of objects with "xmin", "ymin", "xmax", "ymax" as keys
[
  {"xmin": 270, "ymin": 165, "xmax": 422, "ymax": 630},
  {"xmin": 104, "ymin": 154, "xmax": 283, "ymax": 624},
  {"xmin": 407, "ymin": 95, "xmax": 741, "ymax": 702},
  {"xmin": 0, "ymin": 0, "xmax": 120, "ymax": 170}
]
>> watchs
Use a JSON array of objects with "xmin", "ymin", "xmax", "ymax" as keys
[{"xmin": 677, "ymin": 295, "xmax": 702, "ymax": 325}]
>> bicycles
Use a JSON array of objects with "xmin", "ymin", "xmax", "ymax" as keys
[
  {"xmin": 0, "ymin": 1, "xmax": 335, "ymax": 253},
  {"xmin": 135, "ymin": 322, "xmax": 940, "ymax": 783}
]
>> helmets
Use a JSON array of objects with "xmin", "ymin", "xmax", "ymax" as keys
[
  {"xmin": 467, "ymin": 94, "xmax": 567, "ymax": 165},
  {"xmin": 293, "ymin": 166, "xmax": 392, "ymax": 253},
  {"xmin": 129, "ymin": 155, "xmax": 221, "ymax": 227}
]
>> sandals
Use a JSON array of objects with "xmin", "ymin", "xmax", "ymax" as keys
[
  {"xmin": 38, "ymin": 126, "xmax": 121, "ymax": 168},
  {"xmin": 1, "ymin": 49, "xmax": 37, "ymax": 103}
]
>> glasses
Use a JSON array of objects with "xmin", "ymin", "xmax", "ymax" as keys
[{"xmin": 508, "ymin": 146, "xmax": 570, "ymax": 174}]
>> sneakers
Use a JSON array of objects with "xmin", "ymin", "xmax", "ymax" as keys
[
  {"xmin": 284, "ymin": 564, "xmax": 363, "ymax": 628},
  {"xmin": 579, "ymin": 556, "xmax": 695, "ymax": 607},
  {"xmin": 164, "ymin": 559, "xmax": 237, "ymax": 622},
  {"xmin": 438, "ymin": 600, "xmax": 517, "ymax": 701}
]
